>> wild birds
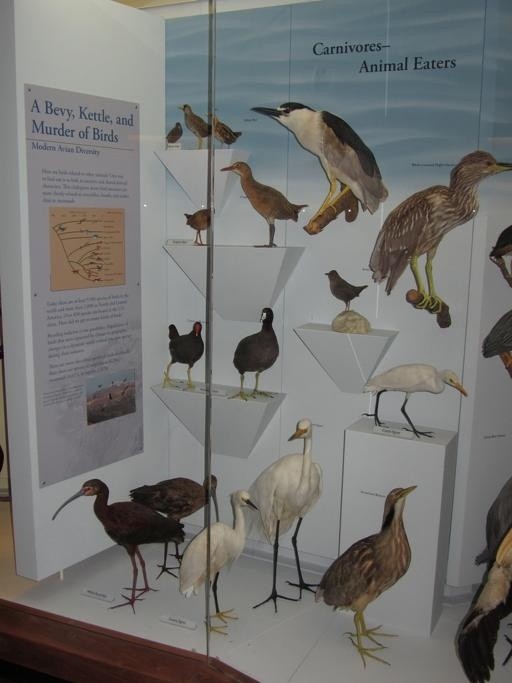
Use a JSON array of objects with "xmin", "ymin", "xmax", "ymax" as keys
[
  {"xmin": 455, "ymin": 224, "xmax": 511, "ymax": 683},
  {"xmin": 52, "ymin": 478, "xmax": 186, "ymax": 614},
  {"xmin": 181, "ymin": 204, "xmax": 215, "ymax": 247},
  {"xmin": 242, "ymin": 416, "xmax": 325, "ymax": 615},
  {"xmin": 316, "ymin": 484, "xmax": 417, "ymax": 671},
  {"xmin": 248, "ymin": 101, "xmax": 390, "ymax": 223},
  {"xmin": 371, "ymin": 149, "xmax": 512, "ymax": 327},
  {"xmin": 216, "ymin": 160, "xmax": 310, "ymax": 248},
  {"xmin": 179, "ymin": 488, "xmax": 261, "ymax": 640},
  {"xmin": 129, "ymin": 473, "xmax": 220, "ymax": 581},
  {"xmin": 165, "ymin": 97, "xmax": 244, "ymax": 150},
  {"xmin": 360, "ymin": 363, "xmax": 468, "ymax": 436},
  {"xmin": 165, "ymin": 321, "xmax": 206, "ymax": 391},
  {"xmin": 227, "ymin": 306, "xmax": 282, "ymax": 404},
  {"xmin": 324, "ymin": 269, "xmax": 369, "ymax": 312}
]
